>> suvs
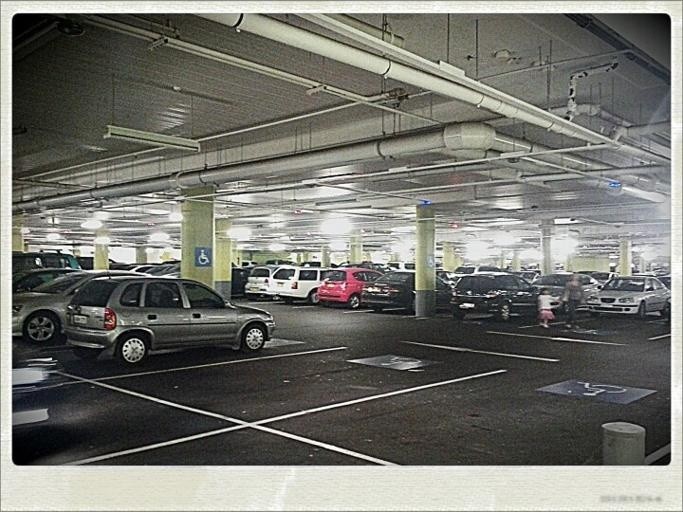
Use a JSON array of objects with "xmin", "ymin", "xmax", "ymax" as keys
[{"xmin": 12, "ymin": 248, "xmax": 81, "ymax": 274}]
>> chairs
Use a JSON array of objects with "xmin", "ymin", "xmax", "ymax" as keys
[{"xmin": 136, "ymin": 288, "xmax": 176, "ymax": 309}]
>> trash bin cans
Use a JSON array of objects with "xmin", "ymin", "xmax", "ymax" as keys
[{"xmin": 600, "ymin": 421, "xmax": 646, "ymax": 465}]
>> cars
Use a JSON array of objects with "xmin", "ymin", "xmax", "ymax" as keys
[
  {"xmin": 12, "ymin": 268, "xmax": 153, "ymax": 345},
  {"xmin": 63, "ymin": 275, "xmax": 278, "ymax": 368}
]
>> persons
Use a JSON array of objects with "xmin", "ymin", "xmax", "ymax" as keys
[
  {"xmin": 537, "ymin": 287, "xmax": 560, "ymax": 329},
  {"xmin": 560, "ymin": 272, "xmax": 586, "ymax": 330}
]
